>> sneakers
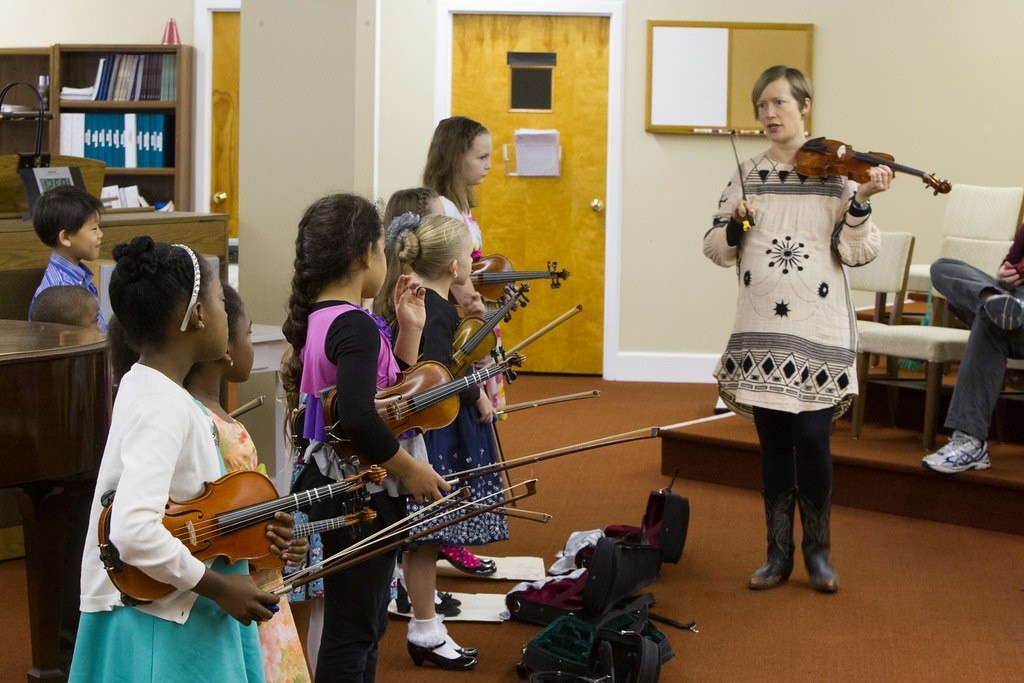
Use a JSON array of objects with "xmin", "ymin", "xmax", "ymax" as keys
[
  {"xmin": 984, "ymin": 294, "xmax": 1024, "ymax": 330},
  {"xmin": 920, "ymin": 429, "xmax": 992, "ymax": 473}
]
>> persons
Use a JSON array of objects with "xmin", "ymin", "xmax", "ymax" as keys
[
  {"xmin": 281, "ymin": 193, "xmax": 453, "ymax": 683},
  {"xmin": 396, "ymin": 214, "xmax": 511, "ymax": 671},
  {"xmin": 29, "ymin": 285, "xmax": 101, "ymax": 330},
  {"xmin": 921, "ymin": 193, "xmax": 1024, "ymax": 474},
  {"xmin": 65, "ymin": 235, "xmax": 294, "ymax": 683},
  {"xmin": 704, "ymin": 65, "xmax": 893, "ymax": 593},
  {"xmin": 29, "ymin": 185, "xmax": 106, "ymax": 331},
  {"xmin": 422, "ymin": 116, "xmax": 508, "ymax": 421},
  {"xmin": 107, "ymin": 283, "xmax": 311, "ymax": 683},
  {"xmin": 370, "ymin": 188, "xmax": 497, "ymax": 575}
]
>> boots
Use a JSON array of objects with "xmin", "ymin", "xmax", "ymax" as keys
[
  {"xmin": 797, "ymin": 483, "xmax": 838, "ymax": 593},
  {"xmin": 749, "ymin": 487, "xmax": 796, "ymax": 588}
]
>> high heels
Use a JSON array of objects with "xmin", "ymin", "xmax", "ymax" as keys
[
  {"xmin": 406, "ymin": 636, "xmax": 480, "ymax": 671},
  {"xmin": 394, "ymin": 581, "xmax": 462, "ymax": 618}
]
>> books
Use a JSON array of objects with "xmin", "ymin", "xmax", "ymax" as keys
[
  {"xmin": 59, "ymin": 53, "xmax": 177, "ymax": 101},
  {"xmin": 101, "ymin": 185, "xmax": 141, "ymax": 208},
  {"xmin": 60, "ymin": 113, "xmax": 166, "ymax": 168}
]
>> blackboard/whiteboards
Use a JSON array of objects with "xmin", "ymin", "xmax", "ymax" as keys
[{"xmin": 644, "ymin": 20, "xmax": 814, "ymax": 139}]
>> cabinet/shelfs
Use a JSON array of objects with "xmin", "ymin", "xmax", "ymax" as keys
[{"xmin": 0, "ymin": 44, "xmax": 197, "ymax": 211}]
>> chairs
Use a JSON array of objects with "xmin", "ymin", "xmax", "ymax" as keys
[
  {"xmin": 872, "ymin": 184, "xmax": 1024, "ymax": 367},
  {"xmin": 829, "ymin": 231, "xmax": 916, "ymax": 437},
  {"xmin": 851, "ymin": 237, "xmax": 1016, "ymax": 452}
]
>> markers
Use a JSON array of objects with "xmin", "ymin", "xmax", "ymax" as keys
[{"xmin": 686, "ymin": 126, "xmax": 809, "ymax": 137}]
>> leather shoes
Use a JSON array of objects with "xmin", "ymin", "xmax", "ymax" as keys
[{"xmin": 437, "ymin": 544, "xmax": 496, "ymax": 576}]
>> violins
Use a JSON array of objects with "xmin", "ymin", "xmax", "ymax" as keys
[
  {"xmin": 470, "ymin": 253, "xmax": 570, "ymax": 301},
  {"xmin": 291, "ymin": 404, "xmax": 310, "ymax": 457},
  {"xmin": 416, "ymin": 282, "xmax": 530, "ymax": 380},
  {"xmin": 323, "ymin": 345, "xmax": 528, "ymax": 467},
  {"xmin": 97, "ymin": 454, "xmax": 387, "ymax": 607},
  {"xmin": 793, "ymin": 137, "xmax": 953, "ymax": 197},
  {"xmin": 247, "ymin": 506, "xmax": 378, "ymax": 574}
]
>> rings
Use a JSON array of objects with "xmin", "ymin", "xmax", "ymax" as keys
[{"xmin": 876, "ymin": 179, "xmax": 883, "ymax": 182}]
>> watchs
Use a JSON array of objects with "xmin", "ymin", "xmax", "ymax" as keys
[{"xmin": 848, "ymin": 191, "xmax": 871, "ymax": 211}]
